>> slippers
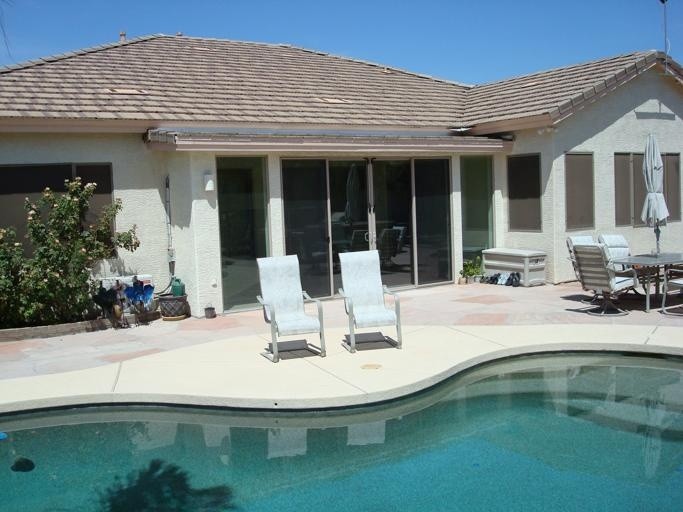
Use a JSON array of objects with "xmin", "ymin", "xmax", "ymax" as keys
[{"xmin": 480, "ymin": 272, "xmax": 521, "ymax": 287}]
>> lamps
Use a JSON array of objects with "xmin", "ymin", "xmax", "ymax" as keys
[{"xmin": 204, "ymin": 174, "xmax": 214, "ymax": 191}]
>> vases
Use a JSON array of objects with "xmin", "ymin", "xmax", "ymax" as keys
[
  {"xmin": 204, "ymin": 306, "xmax": 215, "ymax": 318},
  {"xmin": 159, "ymin": 293, "xmax": 189, "ymax": 321}
]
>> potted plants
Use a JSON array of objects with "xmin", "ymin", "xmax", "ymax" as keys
[{"xmin": 459, "ymin": 254, "xmax": 482, "ymax": 285}]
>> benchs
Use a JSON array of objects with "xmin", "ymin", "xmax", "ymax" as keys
[{"xmin": 481, "ymin": 248, "xmax": 546, "ymax": 287}]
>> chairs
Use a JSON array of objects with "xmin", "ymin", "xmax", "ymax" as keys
[
  {"xmin": 662, "ymin": 269, "xmax": 683, "ymax": 317},
  {"xmin": 265, "ymin": 427, "xmax": 308, "ymax": 459},
  {"xmin": 255, "ymin": 254, "xmax": 326, "ymax": 363},
  {"xmin": 597, "ymin": 233, "xmax": 661, "ymax": 300},
  {"xmin": 566, "ymin": 233, "xmax": 646, "ymax": 302},
  {"xmin": 301, "ymin": 224, "xmax": 406, "ymax": 279},
  {"xmin": 346, "ymin": 420, "xmax": 387, "ymax": 447},
  {"xmin": 573, "ymin": 243, "xmax": 640, "ymax": 317},
  {"xmin": 338, "ymin": 248, "xmax": 403, "ymax": 353}
]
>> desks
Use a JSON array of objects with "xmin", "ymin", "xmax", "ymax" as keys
[{"xmin": 611, "ymin": 252, "xmax": 683, "ymax": 313}]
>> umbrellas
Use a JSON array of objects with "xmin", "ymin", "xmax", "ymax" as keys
[
  {"xmin": 640, "ymin": 131, "xmax": 670, "ymax": 255},
  {"xmin": 345, "ymin": 163, "xmax": 362, "ymax": 222},
  {"xmin": 640, "ymin": 385, "xmax": 666, "ymax": 480}
]
